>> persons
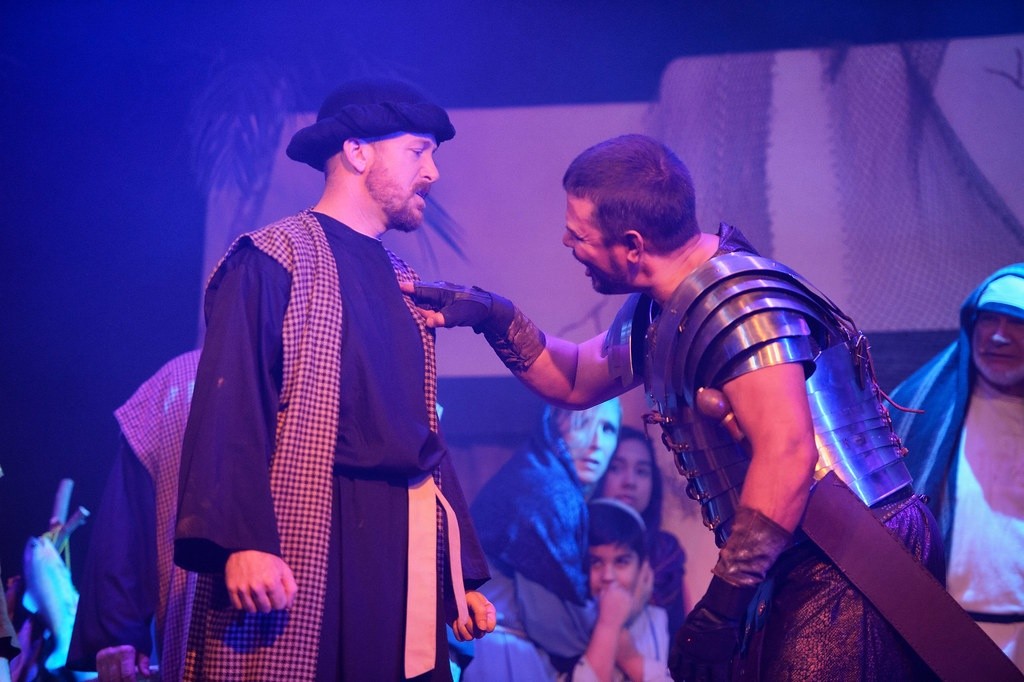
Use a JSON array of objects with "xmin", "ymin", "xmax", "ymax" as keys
[
  {"xmin": 174, "ymin": 74, "xmax": 499, "ymax": 682},
  {"xmin": 581, "ymin": 426, "xmax": 689, "ymax": 638},
  {"xmin": 400, "ymin": 134, "xmax": 947, "ymax": 682},
  {"xmin": 442, "ymin": 396, "xmax": 653, "ymax": 681},
  {"xmin": 84, "ymin": 347, "xmax": 206, "ymax": 682},
  {"xmin": 564, "ymin": 500, "xmax": 674, "ymax": 682},
  {"xmin": 884, "ymin": 262, "xmax": 1024, "ymax": 682}
]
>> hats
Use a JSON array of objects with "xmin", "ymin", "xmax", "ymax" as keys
[{"xmin": 284, "ymin": 78, "xmax": 458, "ymax": 165}]
